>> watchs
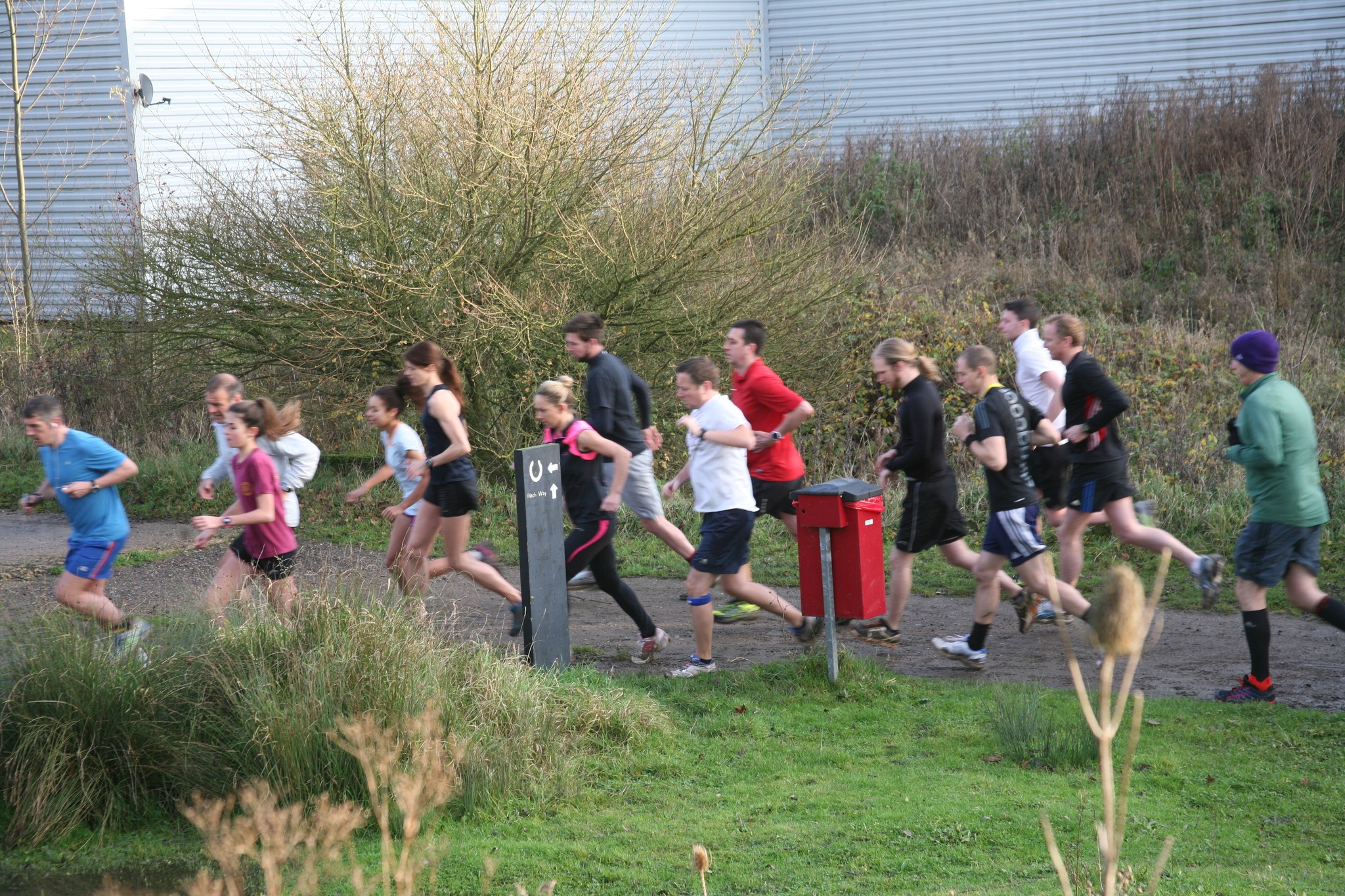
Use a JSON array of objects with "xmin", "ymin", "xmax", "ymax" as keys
[
  {"xmin": 425, "ymin": 455, "xmax": 434, "ymax": 470},
  {"xmin": 771, "ymin": 431, "xmax": 782, "ymax": 441},
  {"xmin": 90, "ymin": 480, "xmax": 100, "ymax": 493},
  {"xmin": 223, "ymin": 515, "xmax": 231, "ymax": 526}
]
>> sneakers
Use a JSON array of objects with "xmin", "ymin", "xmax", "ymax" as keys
[
  {"xmin": 1190, "ymin": 551, "xmax": 1225, "ymax": 611},
  {"xmin": 1032, "ymin": 601, "xmax": 1073, "ymax": 625},
  {"xmin": 932, "ymin": 632, "xmax": 987, "ymax": 670},
  {"xmin": 664, "ymin": 656, "xmax": 717, "ymax": 677},
  {"xmin": 788, "ymin": 614, "xmax": 822, "ymax": 642},
  {"xmin": 1011, "ymin": 589, "xmax": 1042, "ymax": 634},
  {"xmin": 712, "ymin": 600, "xmax": 760, "ymax": 623},
  {"xmin": 679, "ymin": 575, "xmax": 723, "ymax": 600},
  {"xmin": 835, "ymin": 616, "xmax": 851, "ymax": 623},
  {"xmin": 631, "ymin": 626, "xmax": 672, "ymax": 664},
  {"xmin": 849, "ymin": 619, "xmax": 902, "ymax": 648},
  {"xmin": 1134, "ymin": 499, "xmax": 1161, "ymax": 529},
  {"xmin": 1213, "ymin": 674, "xmax": 1276, "ymax": 704}
]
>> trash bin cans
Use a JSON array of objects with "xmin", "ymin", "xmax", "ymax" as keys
[{"xmin": 791, "ymin": 479, "xmax": 889, "ymax": 619}]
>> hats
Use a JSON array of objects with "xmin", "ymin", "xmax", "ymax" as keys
[{"xmin": 1231, "ymin": 330, "xmax": 1280, "ymax": 375}]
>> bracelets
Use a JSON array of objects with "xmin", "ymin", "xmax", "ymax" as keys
[
  {"xmin": 964, "ymin": 434, "xmax": 978, "ymax": 449},
  {"xmin": 1082, "ymin": 422, "xmax": 1092, "ymax": 436},
  {"xmin": 30, "ymin": 493, "xmax": 43, "ymax": 504},
  {"xmin": 699, "ymin": 428, "xmax": 706, "ymax": 440}
]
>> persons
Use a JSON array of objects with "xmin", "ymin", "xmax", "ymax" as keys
[
  {"xmin": 402, "ymin": 341, "xmax": 525, "ymax": 636},
  {"xmin": 16, "ymin": 395, "xmax": 153, "ymax": 667},
  {"xmin": 532, "ymin": 374, "xmax": 671, "ymax": 665},
  {"xmin": 342, "ymin": 379, "xmax": 501, "ymax": 600},
  {"xmin": 712, "ymin": 320, "xmax": 815, "ymax": 624},
  {"xmin": 1215, "ymin": 330, "xmax": 1345, "ymax": 703},
  {"xmin": 188, "ymin": 372, "xmax": 324, "ymax": 626},
  {"xmin": 658, "ymin": 355, "xmax": 824, "ymax": 678},
  {"xmin": 996, "ymin": 298, "xmax": 1151, "ymax": 607},
  {"xmin": 564, "ymin": 313, "xmax": 720, "ymax": 601},
  {"xmin": 849, "ymin": 338, "xmax": 1039, "ymax": 649},
  {"xmin": 930, "ymin": 345, "xmax": 1093, "ymax": 665},
  {"xmin": 1039, "ymin": 313, "xmax": 1223, "ymax": 626}
]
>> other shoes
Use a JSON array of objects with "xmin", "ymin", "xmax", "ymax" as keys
[
  {"xmin": 134, "ymin": 648, "xmax": 152, "ymax": 668},
  {"xmin": 477, "ymin": 542, "xmax": 502, "ymax": 575},
  {"xmin": 106, "ymin": 615, "xmax": 151, "ymax": 660},
  {"xmin": 569, "ymin": 568, "xmax": 597, "ymax": 586},
  {"xmin": 510, "ymin": 605, "xmax": 523, "ymax": 636}
]
